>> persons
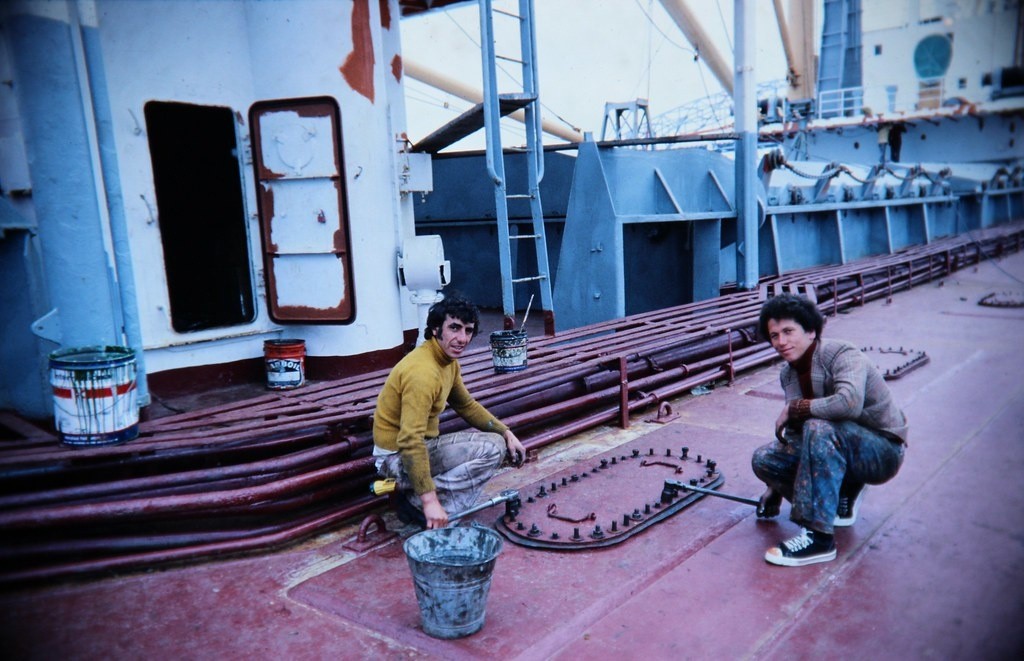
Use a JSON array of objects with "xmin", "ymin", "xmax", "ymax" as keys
[
  {"xmin": 373, "ymin": 294, "xmax": 526, "ymax": 529},
  {"xmin": 752, "ymin": 293, "xmax": 909, "ymax": 566}
]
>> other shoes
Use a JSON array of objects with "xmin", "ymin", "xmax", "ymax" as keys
[{"xmin": 396, "ymin": 501, "xmax": 427, "ymax": 528}]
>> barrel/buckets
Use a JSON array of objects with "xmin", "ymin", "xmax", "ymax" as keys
[
  {"xmin": 47, "ymin": 345, "xmax": 140, "ymax": 444},
  {"xmin": 403, "ymin": 528, "xmax": 503, "ymax": 640},
  {"xmin": 264, "ymin": 339, "xmax": 305, "ymax": 390},
  {"xmin": 490, "ymin": 330, "xmax": 529, "ymax": 373}
]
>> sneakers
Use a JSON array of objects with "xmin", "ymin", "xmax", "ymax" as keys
[
  {"xmin": 834, "ymin": 498, "xmax": 856, "ymax": 527},
  {"xmin": 765, "ymin": 527, "xmax": 837, "ymax": 566}
]
